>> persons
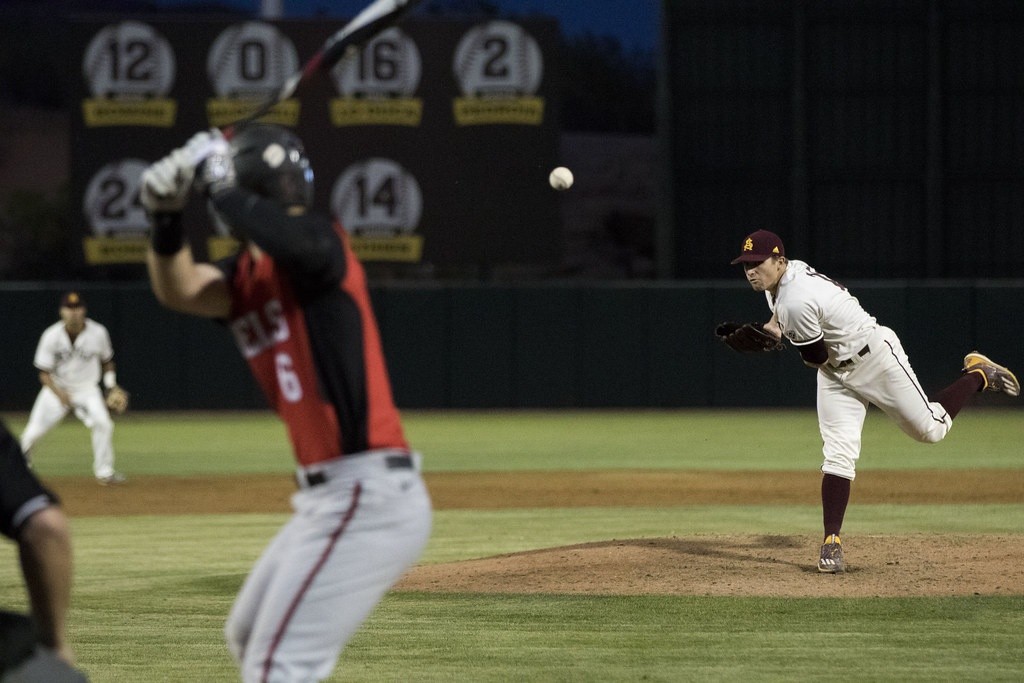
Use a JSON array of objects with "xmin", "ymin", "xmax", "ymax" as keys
[
  {"xmin": 0, "ymin": 419, "xmax": 91, "ymax": 683},
  {"xmin": 715, "ymin": 229, "xmax": 1020, "ymax": 574},
  {"xmin": 140, "ymin": 121, "xmax": 432, "ymax": 683},
  {"xmin": 20, "ymin": 290, "xmax": 131, "ymax": 486}
]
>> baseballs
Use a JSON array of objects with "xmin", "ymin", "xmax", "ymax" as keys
[{"xmin": 548, "ymin": 166, "xmax": 575, "ymax": 191}]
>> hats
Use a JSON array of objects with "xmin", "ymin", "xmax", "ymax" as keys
[
  {"xmin": 60, "ymin": 289, "xmax": 85, "ymax": 308},
  {"xmin": 730, "ymin": 229, "xmax": 784, "ymax": 264}
]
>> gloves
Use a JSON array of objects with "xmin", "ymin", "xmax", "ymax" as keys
[
  {"xmin": 180, "ymin": 127, "xmax": 238, "ymax": 197},
  {"xmin": 138, "ymin": 150, "xmax": 194, "ymax": 212}
]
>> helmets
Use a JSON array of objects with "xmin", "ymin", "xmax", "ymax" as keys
[{"xmin": 206, "ymin": 121, "xmax": 315, "ymax": 227}]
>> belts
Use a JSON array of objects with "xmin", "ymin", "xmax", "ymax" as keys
[
  {"xmin": 827, "ymin": 344, "xmax": 869, "ymax": 368},
  {"xmin": 293, "ymin": 454, "xmax": 414, "ymax": 487}
]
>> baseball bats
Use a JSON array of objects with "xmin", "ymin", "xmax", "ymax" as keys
[{"xmin": 227, "ymin": 0, "xmax": 410, "ymax": 134}]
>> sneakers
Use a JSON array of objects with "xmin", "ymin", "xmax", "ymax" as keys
[
  {"xmin": 818, "ymin": 533, "xmax": 843, "ymax": 573},
  {"xmin": 961, "ymin": 351, "xmax": 1020, "ymax": 397}
]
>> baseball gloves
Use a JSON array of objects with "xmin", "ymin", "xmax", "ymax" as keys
[
  {"xmin": 108, "ymin": 387, "xmax": 129, "ymax": 413},
  {"xmin": 711, "ymin": 321, "xmax": 778, "ymax": 356}
]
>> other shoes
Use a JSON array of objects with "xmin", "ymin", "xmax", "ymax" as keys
[{"xmin": 98, "ymin": 471, "xmax": 126, "ymax": 486}]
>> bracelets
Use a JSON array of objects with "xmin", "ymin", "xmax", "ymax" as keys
[
  {"xmin": 151, "ymin": 209, "xmax": 187, "ymax": 259},
  {"xmin": 103, "ymin": 370, "xmax": 116, "ymax": 388}
]
